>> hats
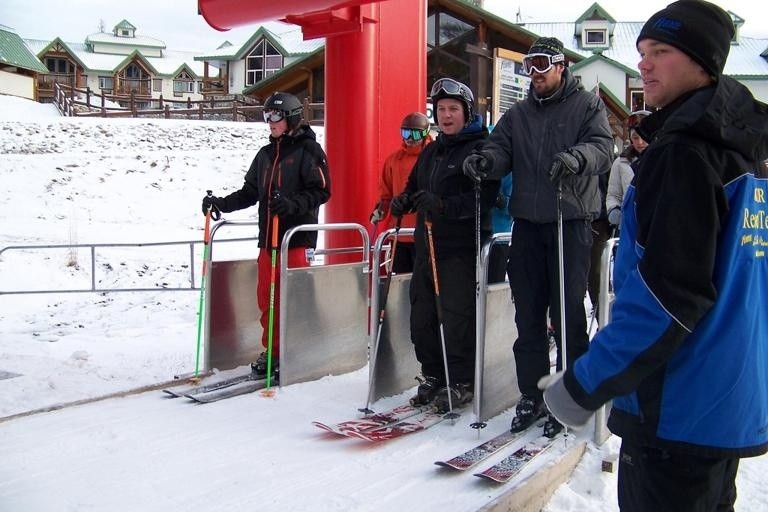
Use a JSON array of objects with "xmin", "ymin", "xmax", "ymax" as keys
[
  {"xmin": 527, "ymin": 36, "xmax": 566, "ymax": 66},
  {"xmin": 636, "ymin": 0, "xmax": 736, "ymax": 83}
]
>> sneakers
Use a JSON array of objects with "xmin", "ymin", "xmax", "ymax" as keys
[
  {"xmin": 252, "ymin": 353, "xmax": 280, "ymax": 381},
  {"xmin": 436, "ymin": 382, "xmax": 474, "ymax": 408},
  {"xmin": 417, "ymin": 376, "xmax": 439, "ymax": 406},
  {"xmin": 517, "ymin": 394, "xmax": 544, "ymax": 420}
]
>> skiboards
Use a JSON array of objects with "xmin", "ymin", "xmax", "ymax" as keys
[
  {"xmin": 310, "ymin": 402, "xmax": 468, "ymax": 443},
  {"xmin": 435, "ymin": 419, "xmax": 570, "ymax": 484},
  {"xmin": 163, "ymin": 373, "xmax": 279, "ymax": 404}
]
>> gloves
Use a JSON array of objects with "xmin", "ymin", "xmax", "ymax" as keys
[
  {"xmin": 607, "ymin": 208, "xmax": 622, "ymax": 226},
  {"xmin": 546, "ymin": 150, "xmax": 582, "ymax": 185},
  {"xmin": 368, "ymin": 208, "xmax": 386, "ymax": 224},
  {"xmin": 461, "ymin": 152, "xmax": 491, "ymax": 183},
  {"xmin": 201, "ymin": 194, "xmax": 226, "ymax": 216},
  {"xmin": 268, "ymin": 192, "xmax": 298, "ymax": 217},
  {"xmin": 409, "ymin": 189, "xmax": 443, "ymax": 218},
  {"xmin": 390, "ymin": 192, "xmax": 409, "ymax": 216}
]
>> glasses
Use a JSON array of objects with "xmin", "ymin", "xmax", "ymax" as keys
[
  {"xmin": 262, "ymin": 106, "xmax": 304, "ymax": 123},
  {"xmin": 430, "ymin": 78, "xmax": 475, "ymax": 106},
  {"xmin": 400, "ymin": 124, "xmax": 430, "ymax": 141},
  {"xmin": 523, "ymin": 52, "xmax": 565, "ymax": 75}
]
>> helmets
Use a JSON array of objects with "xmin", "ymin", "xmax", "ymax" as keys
[
  {"xmin": 626, "ymin": 109, "xmax": 655, "ymax": 145},
  {"xmin": 430, "ymin": 80, "xmax": 475, "ymax": 129},
  {"xmin": 262, "ymin": 90, "xmax": 303, "ymax": 118},
  {"xmin": 400, "ymin": 112, "xmax": 432, "ymax": 138}
]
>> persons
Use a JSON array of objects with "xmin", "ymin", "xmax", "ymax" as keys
[
  {"xmin": 535, "ymin": 0, "xmax": 768, "ymax": 512},
  {"xmin": 459, "ymin": 36, "xmax": 617, "ymax": 438},
  {"xmin": 202, "ymin": 92, "xmax": 335, "ymax": 381},
  {"xmin": 480, "ymin": 124, "xmax": 514, "ymax": 283},
  {"xmin": 369, "ymin": 110, "xmax": 435, "ymax": 276},
  {"xmin": 389, "ymin": 77, "xmax": 503, "ymax": 415},
  {"xmin": 586, "ymin": 169, "xmax": 613, "ymax": 327},
  {"xmin": 603, "ymin": 108, "xmax": 657, "ymax": 226}
]
